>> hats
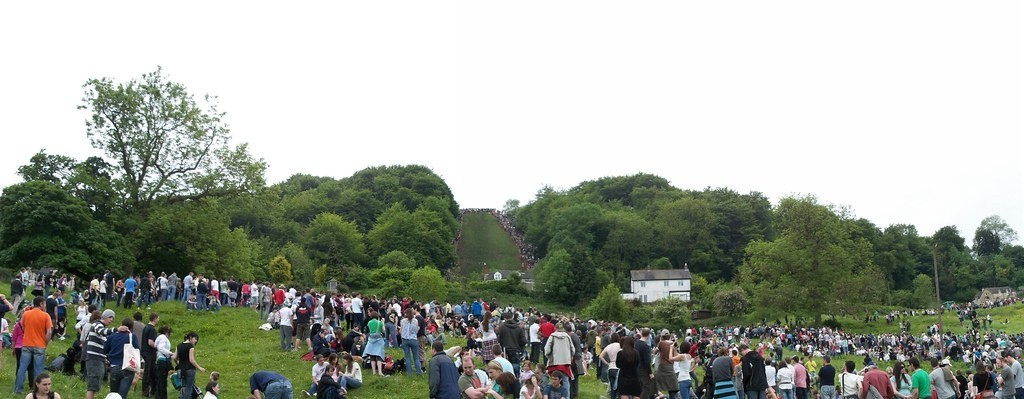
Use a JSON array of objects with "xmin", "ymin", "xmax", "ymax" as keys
[
  {"xmin": 556, "ymin": 323, "xmax": 564, "ymax": 331},
  {"xmin": 101, "ymin": 309, "xmax": 115, "ymax": 320},
  {"xmin": 867, "ymin": 362, "xmax": 878, "ymax": 367},
  {"xmin": 661, "ymin": 329, "xmax": 670, "ymax": 336},
  {"xmin": 941, "ymin": 359, "xmax": 952, "ymax": 366},
  {"xmin": 279, "ymin": 284, "xmax": 285, "ymax": 288}
]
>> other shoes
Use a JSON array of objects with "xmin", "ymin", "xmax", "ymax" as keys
[{"xmin": 301, "ymin": 390, "xmax": 312, "ymax": 397}]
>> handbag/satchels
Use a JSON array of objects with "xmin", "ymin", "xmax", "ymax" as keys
[
  {"xmin": 166, "ymin": 358, "xmax": 174, "ymax": 370},
  {"xmin": 982, "ymin": 389, "xmax": 995, "ymax": 399},
  {"xmin": 844, "ymin": 394, "xmax": 856, "ymax": 399},
  {"xmin": 122, "ymin": 332, "xmax": 140, "ymax": 373}
]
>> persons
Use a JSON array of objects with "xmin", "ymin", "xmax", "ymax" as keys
[
  {"xmin": 452, "ymin": 207, "xmax": 541, "ymax": 280},
  {"xmin": 0, "ymin": 260, "xmax": 1024, "ymax": 399}
]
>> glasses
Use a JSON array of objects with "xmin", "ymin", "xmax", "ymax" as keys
[{"xmin": 901, "ymin": 366, "xmax": 905, "ymax": 369}]
